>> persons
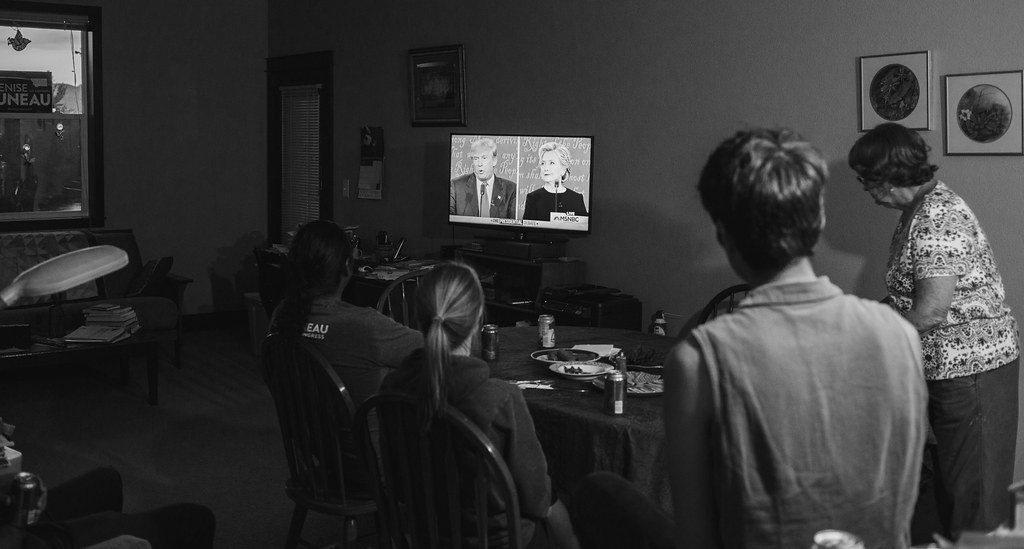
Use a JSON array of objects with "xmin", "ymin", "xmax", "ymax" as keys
[
  {"xmin": 563, "ymin": 130, "xmax": 929, "ymax": 549},
  {"xmin": 267, "ymin": 220, "xmax": 426, "ymax": 491},
  {"xmin": 449, "ymin": 139, "xmax": 517, "ymax": 221},
  {"xmin": 523, "ymin": 143, "xmax": 588, "ymax": 223},
  {"xmin": 849, "ymin": 121, "xmax": 1021, "ymax": 546},
  {"xmin": 383, "ymin": 261, "xmax": 578, "ymax": 549},
  {"xmin": 0, "ymin": 467, "xmax": 216, "ymax": 549}
]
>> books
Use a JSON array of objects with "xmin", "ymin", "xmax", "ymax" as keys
[{"xmin": 64, "ymin": 302, "xmax": 143, "ymax": 344}]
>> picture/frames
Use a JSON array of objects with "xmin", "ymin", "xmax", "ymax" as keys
[
  {"xmin": 945, "ymin": 70, "xmax": 1024, "ymax": 156},
  {"xmin": 408, "ymin": 44, "xmax": 469, "ymax": 125},
  {"xmin": 859, "ymin": 49, "xmax": 932, "ymax": 130}
]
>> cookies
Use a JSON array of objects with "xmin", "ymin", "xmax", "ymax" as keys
[{"xmin": 626, "ymin": 372, "xmax": 664, "ymax": 393}]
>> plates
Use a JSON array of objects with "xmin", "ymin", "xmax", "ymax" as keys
[
  {"xmin": 593, "ymin": 371, "xmax": 664, "ymax": 395},
  {"xmin": 549, "ymin": 362, "xmax": 615, "ymax": 380},
  {"xmin": 610, "ymin": 353, "xmax": 664, "ymax": 368},
  {"xmin": 531, "ymin": 348, "xmax": 601, "ymax": 363}
]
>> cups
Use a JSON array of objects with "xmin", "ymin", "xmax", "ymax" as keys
[{"xmin": 353, "ymin": 248, "xmax": 362, "ymax": 259}]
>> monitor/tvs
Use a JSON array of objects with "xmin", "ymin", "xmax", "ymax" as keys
[{"xmin": 448, "ymin": 132, "xmax": 595, "ymax": 243}]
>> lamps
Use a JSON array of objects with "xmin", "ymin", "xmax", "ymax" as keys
[{"xmin": 0, "ymin": 245, "xmax": 129, "ymax": 306}]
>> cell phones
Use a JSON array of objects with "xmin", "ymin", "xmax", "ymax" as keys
[{"xmin": 392, "ymin": 238, "xmax": 405, "ymax": 258}]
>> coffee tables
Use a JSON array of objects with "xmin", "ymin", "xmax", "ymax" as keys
[{"xmin": 0, "ymin": 332, "xmax": 160, "ymax": 406}]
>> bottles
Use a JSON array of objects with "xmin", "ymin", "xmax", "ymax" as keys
[{"xmin": 616, "ymin": 351, "xmax": 627, "ymax": 373}]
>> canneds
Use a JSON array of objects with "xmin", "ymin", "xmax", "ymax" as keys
[
  {"xmin": 602, "ymin": 369, "xmax": 627, "ymax": 416},
  {"xmin": 481, "ymin": 324, "xmax": 499, "ymax": 361},
  {"xmin": 537, "ymin": 314, "xmax": 555, "ymax": 348},
  {"xmin": 9, "ymin": 472, "xmax": 41, "ymax": 527}
]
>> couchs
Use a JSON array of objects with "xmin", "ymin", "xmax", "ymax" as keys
[{"xmin": 0, "ymin": 222, "xmax": 191, "ymax": 369}]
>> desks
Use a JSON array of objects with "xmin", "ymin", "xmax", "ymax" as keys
[
  {"xmin": 255, "ymin": 252, "xmax": 437, "ymax": 328},
  {"xmin": 472, "ymin": 325, "xmax": 683, "ymax": 515}
]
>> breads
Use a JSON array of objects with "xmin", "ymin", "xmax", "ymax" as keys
[{"xmin": 557, "ymin": 350, "xmax": 575, "ymax": 361}]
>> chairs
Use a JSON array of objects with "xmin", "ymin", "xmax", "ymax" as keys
[
  {"xmin": 344, "ymin": 392, "xmax": 523, "ymax": 549},
  {"xmin": 258, "ymin": 331, "xmax": 390, "ymax": 549},
  {"xmin": 377, "ymin": 269, "xmax": 437, "ymax": 333},
  {"xmin": 696, "ymin": 282, "xmax": 748, "ymax": 328}
]
